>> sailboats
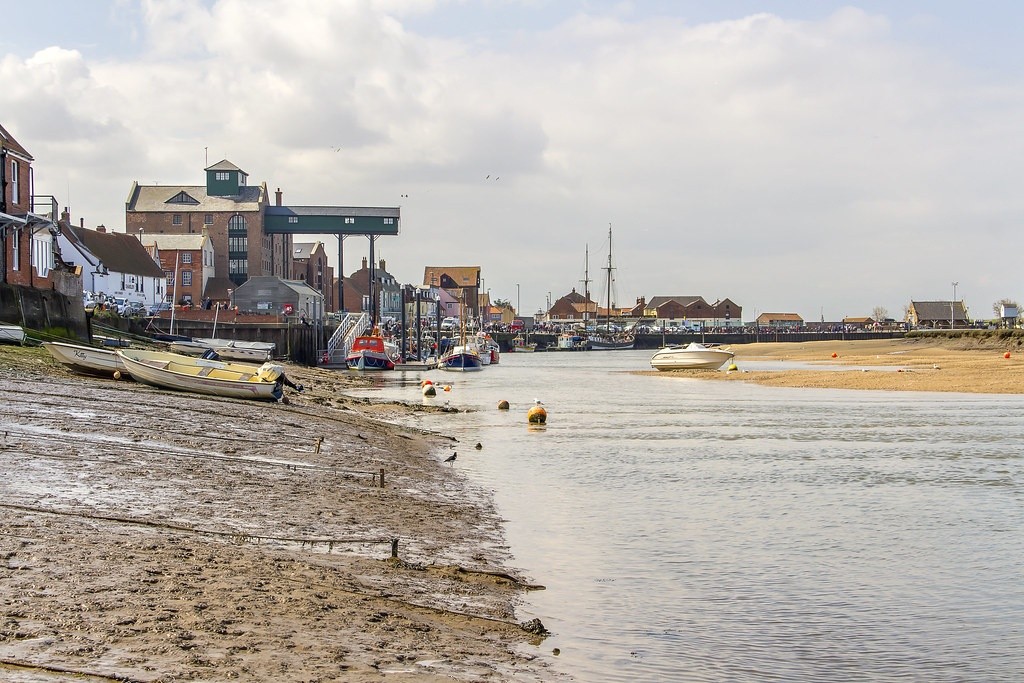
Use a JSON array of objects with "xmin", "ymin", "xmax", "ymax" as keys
[
  {"xmin": 143, "ymin": 252, "xmax": 193, "ymax": 342},
  {"xmin": 580, "ymin": 222, "xmax": 642, "ymax": 350}
]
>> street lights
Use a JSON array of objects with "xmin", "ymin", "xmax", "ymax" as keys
[
  {"xmin": 481, "ymin": 278, "xmax": 485, "ymax": 332},
  {"xmin": 516, "ymin": 283, "xmax": 520, "ymax": 316},
  {"xmin": 487, "ymin": 288, "xmax": 491, "ymax": 320},
  {"xmin": 318, "ymin": 243, "xmax": 325, "ymax": 295},
  {"xmin": 374, "ymin": 262, "xmax": 378, "ymax": 326},
  {"xmin": 951, "ymin": 281, "xmax": 959, "ymax": 329}
]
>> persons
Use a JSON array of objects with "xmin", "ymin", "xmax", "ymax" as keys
[
  {"xmin": 712, "ymin": 324, "xmax": 858, "ymax": 334},
  {"xmin": 235, "ymin": 304, "xmax": 238, "ymax": 315},
  {"xmin": 223, "ymin": 301, "xmax": 228, "ymax": 310},
  {"xmin": 301, "ymin": 314, "xmax": 306, "ymax": 324}
]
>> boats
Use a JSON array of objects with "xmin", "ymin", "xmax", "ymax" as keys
[
  {"xmin": 41, "ymin": 341, "xmax": 130, "ymax": 378},
  {"xmin": 115, "ymin": 347, "xmax": 305, "ymax": 403},
  {"xmin": 380, "ymin": 286, "xmax": 537, "ymax": 373},
  {"xmin": 344, "ymin": 315, "xmax": 402, "ymax": 371},
  {"xmin": 0, "ymin": 323, "xmax": 28, "ymax": 348},
  {"xmin": 537, "ymin": 321, "xmax": 592, "ymax": 353},
  {"xmin": 92, "ymin": 334, "xmax": 131, "ymax": 347},
  {"xmin": 168, "ymin": 301, "xmax": 276, "ymax": 363},
  {"xmin": 650, "ymin": 342, "xmax": 735, "ymax": 370}
]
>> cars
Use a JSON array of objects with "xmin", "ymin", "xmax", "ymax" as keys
[
  {"xmin": 148, "ymin": 302, "xmax": 173, "ymax": 316},
  {"xmin": 83, "ymin": 289, "xmax": 147, "ymax": 318}
]
